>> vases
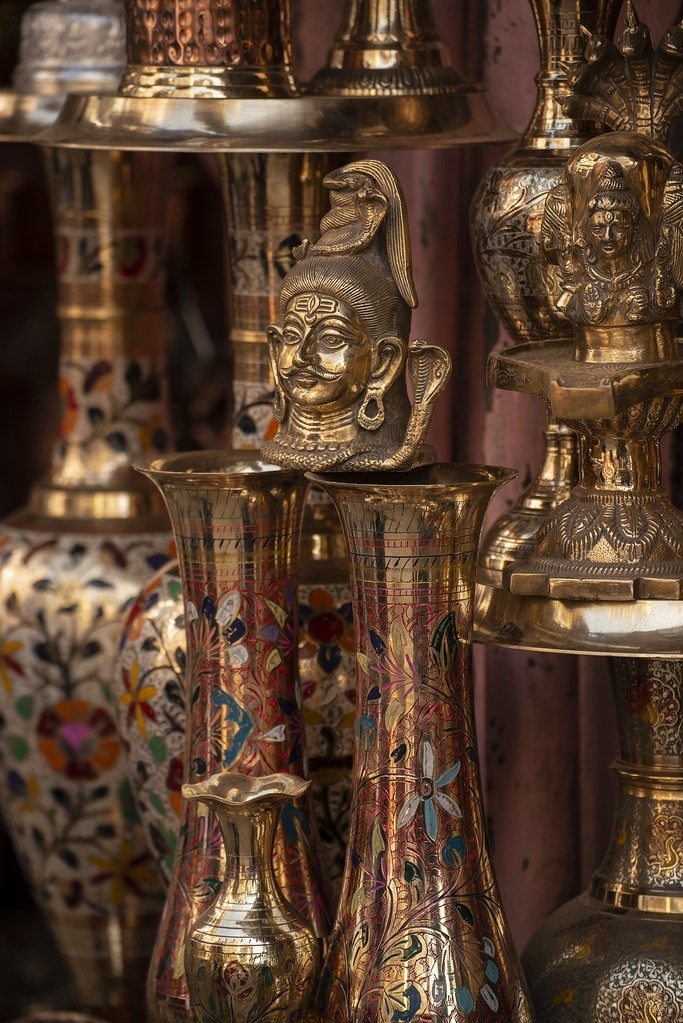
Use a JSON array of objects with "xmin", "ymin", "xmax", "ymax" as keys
[{"xmin": 0, "ymin": 0, "xmax": 683, "ymax": 1023}]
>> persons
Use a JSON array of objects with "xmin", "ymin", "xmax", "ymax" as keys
[
  {"xmin": 262, "ymin": 159, "xmax": 418, "ymax": 474},
  {"xmin": 535, "ymin": 128, "xmax": 681, "ymax": 364}
]
